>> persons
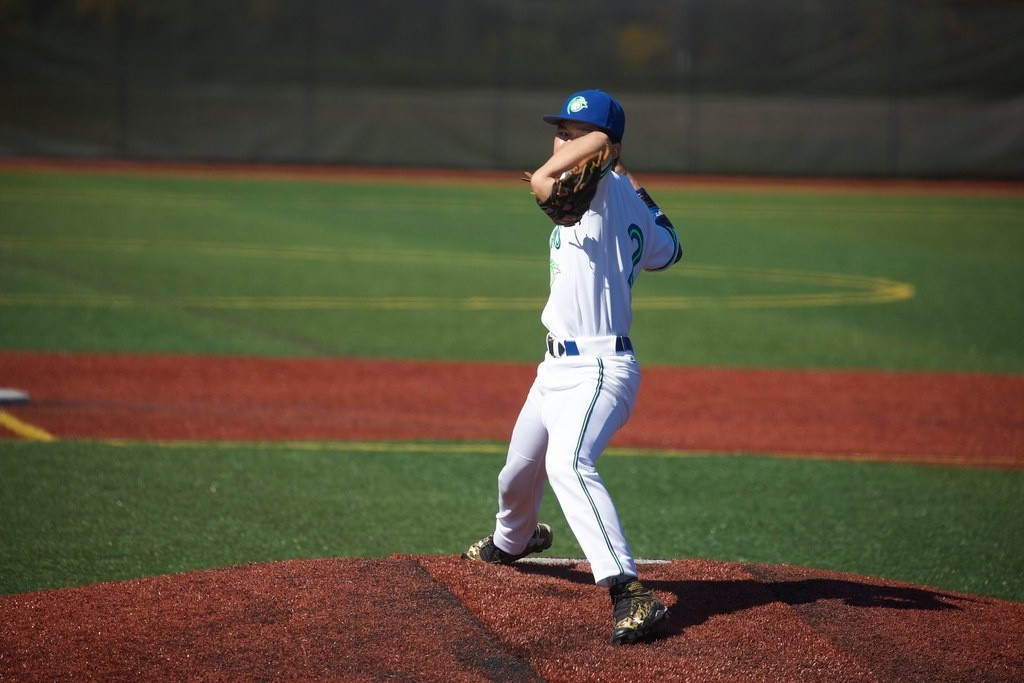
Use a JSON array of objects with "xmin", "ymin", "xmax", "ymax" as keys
[{"xmin": 466, "ymin": 89, "xmax": 683, "ymax": 645}]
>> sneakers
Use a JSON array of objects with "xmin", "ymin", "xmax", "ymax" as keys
[
  {"xmin": 609, "ymin": 577, "xmax": 671, "ymax": 643},
  {"xmin": 461, "ymin": 523, "xmax": 553, "ymax": 564}
]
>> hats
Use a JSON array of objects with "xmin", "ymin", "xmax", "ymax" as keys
[{"xmin": 542, "ymin": 89, "xmax": 625, "ymax": 140}]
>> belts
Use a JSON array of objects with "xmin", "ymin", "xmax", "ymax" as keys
[{"xmin": 545, "ymin": 335, "xmax": 633, "ymax": 358}]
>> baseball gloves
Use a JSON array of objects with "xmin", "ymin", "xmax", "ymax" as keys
[{"xmin": 518, "ymin": 143, "xmax": 613, "ymax": 228}]
